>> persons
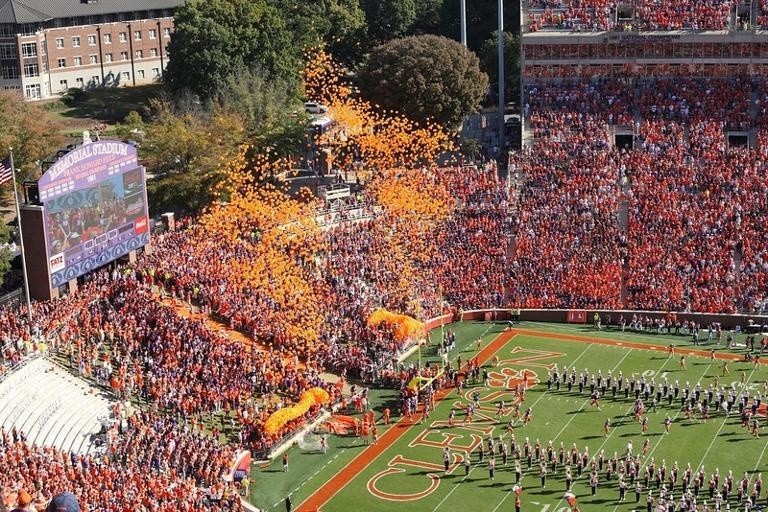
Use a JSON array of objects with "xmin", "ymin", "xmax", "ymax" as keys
[
  {"xmin": 441, "ymin": 434, "xmax": 764, "ymax": 512},
  {"xmin": 500, "ymin": 0, "xmax": 767, "ymax": 323},
  {"xmin": 496, "ymin": 373, "xmax": 532, "ymax": 434},
  {"xmin": 545, "ymin": 311, "xmax": 768, "ymax": 438},
  {"xmin": 318, "ymin": 154, "xmax": 514, "ymax": 444},
  {"xmin": 4, "ymin": 207, "xmax": 346, "ymax": 512}
]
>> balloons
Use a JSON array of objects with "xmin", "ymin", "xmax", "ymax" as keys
[
  {"xmin": 300, "ymin": 20, "xmax": 463, "ymax": 156},
  {"xmin": 204, "ymin": 141, "xmax": 320, "ymax": 212}
]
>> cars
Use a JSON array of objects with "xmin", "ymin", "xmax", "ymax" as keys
[{"xmin": 303, "ymin": 100, "xmax": 327, "ymax": 114}]
